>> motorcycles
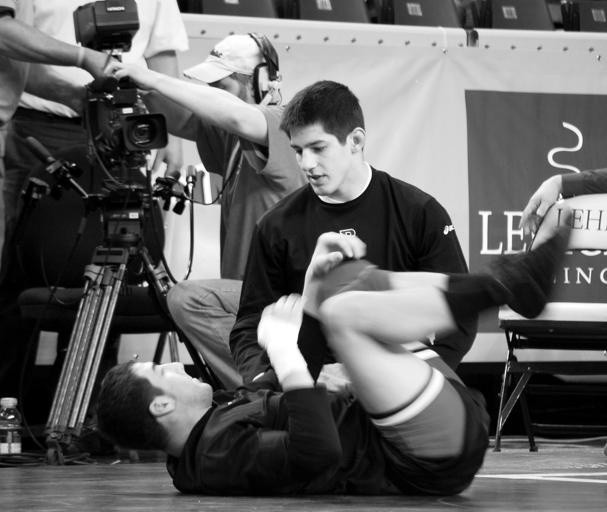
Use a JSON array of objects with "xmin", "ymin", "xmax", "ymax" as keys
[{"xmin": 30, "ymin": 192, "xmax": 224, "ymax": 467}]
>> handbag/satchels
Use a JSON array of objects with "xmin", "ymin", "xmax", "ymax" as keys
[{"xmin": 77, "ymin": 47, "xmax": 86, "ymax": 66}]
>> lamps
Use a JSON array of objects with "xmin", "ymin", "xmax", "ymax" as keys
[
  {"xmin": 287, "ymin": 0, "xmax": 369, "ymax": 24},
  {"xmin": 381, "ymin": 0, "xmax": 461, "ymax": 27},
  {"xmin": 494, "ymin": 191, "xmax": 607, "ymax": 451},
  {"xmin": 481, "ymin": 0, "xmax": 553, "ymax": 31},
  {"xmin": 200, "ymin": 0, "xmax": 276, "ymax": 18},
  {"xmin": 560, "ymin": 0, "xmax": 606, "ymax": 32}
]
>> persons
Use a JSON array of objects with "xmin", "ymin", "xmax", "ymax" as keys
[
  {"xmin": 0, "ymin": 0, "xmax": 188, "ymax": 367},
  {"xmin": 228, "ymin": 79, "xmax": 478, "ymax": 387},
  {"xmin": 518, "ymin": 168, "xmax": 607, "ymax": 236},
  {"xmin": 104, "ymin": 32, "xmax": 311, "ymax": 390},
  {"xmin": 1, "ymin": 0, "xmax": 120, "ymax": 256},
  {"xmin": 96, "ymin": 214, "xmax": 573, "ymax": 497}
]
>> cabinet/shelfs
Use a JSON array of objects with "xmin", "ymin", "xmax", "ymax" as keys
[{"xmin": 0, "ymin": 397, "xmax": 22, "ymax": 456}]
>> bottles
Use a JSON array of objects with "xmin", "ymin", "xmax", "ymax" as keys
[{"xmin": 182, "ymin": 31, "xmax": 276, "ymax": 86}]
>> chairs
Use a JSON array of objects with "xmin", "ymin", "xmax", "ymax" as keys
[{"xmin": 488, "ymin": 211, "xmax": 570, "ymax": 322}]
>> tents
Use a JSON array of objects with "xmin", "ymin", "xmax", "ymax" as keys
[{"xmin": 251, "ymin": 29, "xmax": 281, "ymax": 105}]
